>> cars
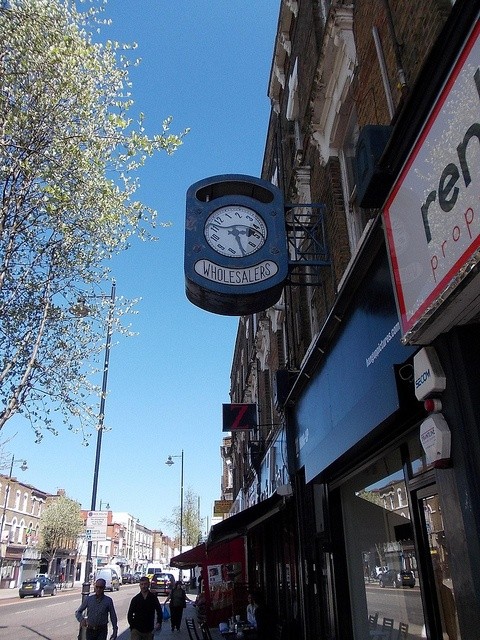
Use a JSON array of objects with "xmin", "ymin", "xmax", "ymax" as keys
[
  {"xmin": 19, "ymin": 577, "xmax": 56, "ymax": 598},
  {"xmin": 121, "ymin": 574, "xmax": 135, "ymax": 584},
  {"xmin": 371, "ymin": 564, "xmax": 388, "ymax": 578},
  {"xmin": 379, "ymin": 568, "xmax": 415, "ymax": 588}
]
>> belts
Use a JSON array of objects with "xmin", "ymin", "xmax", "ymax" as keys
[{"xmin": 88, "ymin": 626, "xmax": 103, "ymax": 630}]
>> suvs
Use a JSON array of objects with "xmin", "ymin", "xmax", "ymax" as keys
[{"xmin": 150, "ymin": 573, "xmax": 175, "ymax": 597}]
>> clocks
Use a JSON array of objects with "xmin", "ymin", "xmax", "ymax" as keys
[{"xmin": 184, "ymin": 173, "xmax": 289, "ymax": 316}]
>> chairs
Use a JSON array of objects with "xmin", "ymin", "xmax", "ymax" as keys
[
  {"xmin": 198, "ymin": 619, "xmax": 214, "ymax": 639},
  {"xmin": 399, "ymin": 622, "xmax": 408, "ymax": 633},
  {"xmin": 369, "ymin": 612, "xmax": 378, "ymax": 624},
  {"xmin": 185, "ymin": 617, "xmax": 200, "ymax": 640},
  {"xmin": 383, "ymin": 617, "xmax": 394, "ymax": 628}
]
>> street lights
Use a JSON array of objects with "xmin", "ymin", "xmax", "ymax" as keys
[
  {"xmin": 100, "ymin": 499, "xmax": 109, "ymax": 512},
  {"xmin": 0, "ymin": 452, "xmax": 28, "ymax": 562},
  {"xmin": 165, "ymin": 451, "xmax": 184, "ymax": 583},
  {"xmin": 68, "ymin": 281, "xmax": 119, "ymax": 639}
]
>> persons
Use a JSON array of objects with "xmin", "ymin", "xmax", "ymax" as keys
[
  {"xmin": 127, "ymin": 577, "xmax": 162, "ymax": 640},
  {"xmin": 246, "ymin": 590, "xmax": 259, "ymax": 630},
  {"xmin": 164, "ymin": 581, "xmax": 191, "ymax": 633},
  {"xmin": 75, "ymin": 578, "xmax": 118, "ymax": 640},
  {"xmin": 254, "ymin": 596, "xmax": 284, "ymax": 638}
]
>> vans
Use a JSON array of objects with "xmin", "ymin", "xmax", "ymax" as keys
[
  {"xmin": 134, "ymin": 571, "xmax": 145, "ymax": 582},
  {"xmin": 145, "ymin": 566, "xmax": 163, "ymax": 576},
  {"xmin": 94, "ymin": 568, "xmax": 119, "ymax": 592}
]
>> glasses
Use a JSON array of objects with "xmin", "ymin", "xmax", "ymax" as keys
[{"xmin": 96, "ymin": 586, "xmax": 104, "ymax": 588}]
